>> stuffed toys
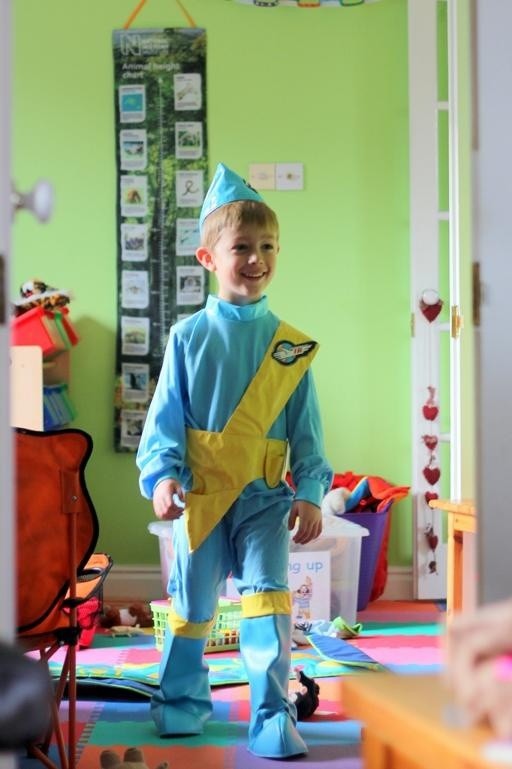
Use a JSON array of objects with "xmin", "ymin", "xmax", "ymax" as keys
[
  {"xmin": 287, "ymin": 669, "xmax": 322, "ymax": 723},
  {"xmin": 98, "ymin": 748, "xmax": 169, "ymax": 769},
  {"xmin": 101, "ymin": 599, "xmax": 153, "ymax": 629}
]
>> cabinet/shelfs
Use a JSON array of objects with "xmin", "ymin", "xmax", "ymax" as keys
[{"xmin": 8, "ymin": 345, "xmax": 74, "ymax": 434}]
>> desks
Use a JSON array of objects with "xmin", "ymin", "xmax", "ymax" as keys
[
  {"xmin": 427, "ymin": 495, "xmax": 477, "ymax": 618},
  {"xmin": 337, "ymin": 668, "xmax": 491, "ymax": 768}
]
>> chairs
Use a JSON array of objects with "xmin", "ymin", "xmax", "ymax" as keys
[{"xmin": 13, "ymin": 426, "xmax": 114, "ymax": 768}]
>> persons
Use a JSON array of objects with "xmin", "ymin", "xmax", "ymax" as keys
[
  {"xmin": 135, "ymin": 163, "xmax": 332, "ymax": 760},
  {"xmin": 292, "ymin": 576, "xmax": 314, "ymax": 619}
]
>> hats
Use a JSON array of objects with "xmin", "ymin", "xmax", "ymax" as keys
[{"xmin": 198, "ymin": 162, "xmax": 264, "ymax": 229}]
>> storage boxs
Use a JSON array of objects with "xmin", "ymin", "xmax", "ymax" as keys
[
  {"xmin": 147, "ymin": 511, "xmax": 370, "ymax": 642},
  {"xmin": 335, "ymin": 498, "xmax": 392, "ymax": 614}
]
